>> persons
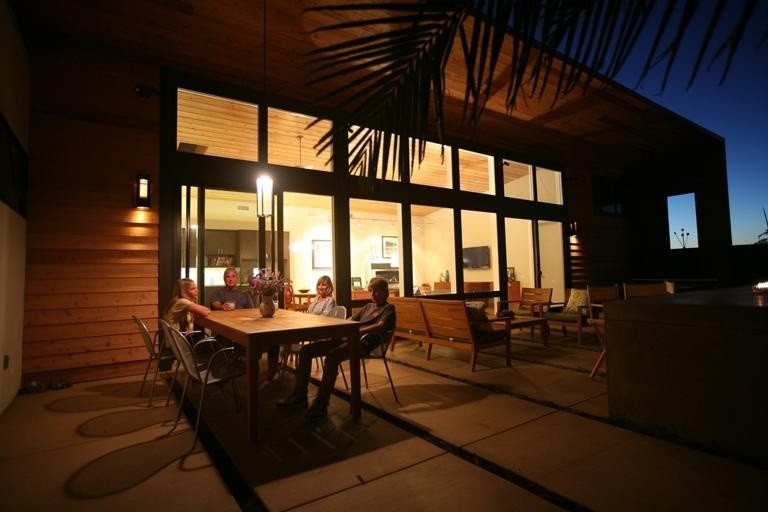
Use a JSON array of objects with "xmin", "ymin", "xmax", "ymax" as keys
[
  {"xmin": 208, "ymin": 266, "xmax": 257, "ymax": 312},
  {"xmin": 272, "ymin": 276, "xmax": 397, "ymax": 421},
  {"xmin": 160, "ymin": 276, "xmax": 231, "ymax": 384},
  {"xmin": 266, "ymin": 274, "xmax": 336, "ymax": 382}
]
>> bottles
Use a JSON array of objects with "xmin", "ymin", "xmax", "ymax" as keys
[
  {"xmin": 439, "ymin": 273, "xmax": 445, "ymax": 283},
  {"xmin": 273, "ymin": 300, "xmax": 278, "ymax": 312},
  {"xmin": 220, "ymin": 247, "xmax": 223, "ymax": 254},
  {"xmin": 445, "ymin": 268, "xmax": 449, "ymax": 283},
  {"xmin": 217, "ymin": 248, "xmax": 220, "ymax": 254}
]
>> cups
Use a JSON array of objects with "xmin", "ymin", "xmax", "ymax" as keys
[{"xmin": 224, "ymin": 298, "xmax": 236, "ymax": 311}]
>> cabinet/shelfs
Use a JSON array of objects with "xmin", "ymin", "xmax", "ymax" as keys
[{"xmin": 179, "ymin": 225, "xmax": 291, "ymax": 311}]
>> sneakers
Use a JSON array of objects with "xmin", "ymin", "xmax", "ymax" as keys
[
  {"xmin": 277, "ymin": 396, "xmax": 308, "ymax": 408},
  {"xmin": 305, "ymin": 398, "xmax": 327, "ymax": 418}
]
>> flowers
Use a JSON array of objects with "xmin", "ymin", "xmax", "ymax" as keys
[{"xmin": 244, "ymin": 265, "xmax": 293, "ymax": 297}]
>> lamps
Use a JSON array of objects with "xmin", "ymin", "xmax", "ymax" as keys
[
  {"xmin": 250, "ymin": 162, "xmax": 279, "ymax": 219},
  {"xmin": 130, "ymin": 171, "xmax": 155, "ymax": 213}
]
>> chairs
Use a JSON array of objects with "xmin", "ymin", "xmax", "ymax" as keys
[
  {"xmin": 384, "ymin": 281, "xmax": 620, "ymax": 383},
  {"xmin": 283, "ymin": 306, "xmax": 349, "ymax": 390},
  {"xmin": 155, "ymin": 328, "xmax": 250, "ymax": 470},
  {"xmin": 133, "ymin": 316, "xmax": 181, "ymax": 403},
  {"xmin": 328, "ymin": 316, "xmax": 397, "ymax": 402},
  {"xmin": 621, "ymin": 280, "xmax": 669, "ymax": 300},
  {"xmin": 162, "ymin": 320, "xmax": 225, "ymax": 407}
]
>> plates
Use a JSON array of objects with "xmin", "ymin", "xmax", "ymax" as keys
[{"xmin": 298, "ymin": 288, "xmax": 309, "ymax": 293}]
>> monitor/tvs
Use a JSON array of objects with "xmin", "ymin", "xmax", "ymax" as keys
[{"xmin": 463, "ymin": 246, "xmax": 490, "ymax": 269}]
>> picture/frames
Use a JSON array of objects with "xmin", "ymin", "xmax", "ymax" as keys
[
  {"xmin": 380, "ymin": 234, "xmax": 400, "ymax": 259},
  {"xmin": 309, "ymin": 239, "xmax": 336, "ymax": 271}
]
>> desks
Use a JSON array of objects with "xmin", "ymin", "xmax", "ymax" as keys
[{"xmin": 191, "ymin": 308, "xmax": 361, "ymax": 452}]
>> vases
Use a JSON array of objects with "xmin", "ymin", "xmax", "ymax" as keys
[{"xmin": 259, "ymin": 294, "xmax": 276, "ymax": 318}]
